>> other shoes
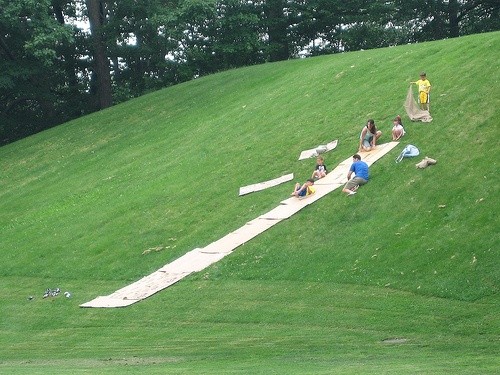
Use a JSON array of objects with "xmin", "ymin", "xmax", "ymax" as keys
[
  {"xmin": 347, "ymin": 192, "xmax": 354, "ymax": 197},
  {"xmin": 290, "ymin": 191, "xmax": 299, "ymax": 196}
]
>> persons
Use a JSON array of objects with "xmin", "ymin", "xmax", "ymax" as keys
[
  {"xmin": 357, "ymin": 119, "xmax": 382, "ymax": 152},
  {"xmin": 410, "ymin": 72, "xmax": 431, "ymax": 113},
  {"xmin": 311, "ymin": 156, "xmax": 328, "ymax": 179},
  {"xmin": 290, "ymin": 178, "xmax": 315, "ymax": 198},
  {"xmin": 341, "ymin": 154, "xmax": 369, "ymax": 196},
  {"xmin": 392, "ymin": 117, "xmax": 404, "ymax": 141}
]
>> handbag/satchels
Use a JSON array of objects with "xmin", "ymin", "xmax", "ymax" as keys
[{"xmin": 396, "ymin": 144, "xmax": 420, "ymax": 163}]
